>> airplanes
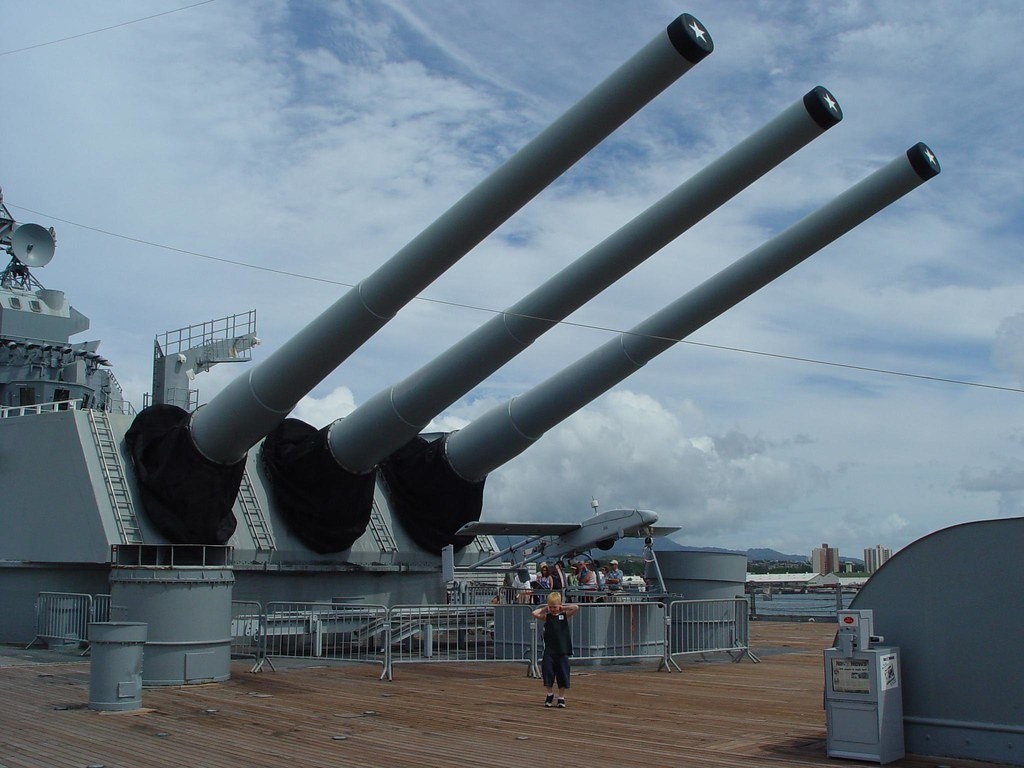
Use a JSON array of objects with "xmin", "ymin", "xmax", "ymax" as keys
[{"xmin": 455, "ymin": 493, "xmax": 682, "ymax": 559}]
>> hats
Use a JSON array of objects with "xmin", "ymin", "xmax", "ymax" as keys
[
  {"xmin": 584, "ymin": 560, "xmax": 592, "ymax": 564},
  {"xmin": 609, "ymin": 560, "xmax": 618, "ymax": 564},
  {"xmin": 570, "ymin": 566, "xmax": 577, "ymax": 569}
]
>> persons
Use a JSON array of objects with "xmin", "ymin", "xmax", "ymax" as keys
[
  {"xmin": 503, "ymin": 558, "xmax": 624, "ymax": 604},
  {"xmin": 531, "ymin": 591, "xmax": 580, "ymax": 707}
]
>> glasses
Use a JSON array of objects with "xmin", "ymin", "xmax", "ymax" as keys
[
  {"xmin": 580, "ymin": 564, "xmax": 584, "ymax": 565},
  {"xmin": 601, "ymin": 569, "xmax": 605, "ymax": 571}
]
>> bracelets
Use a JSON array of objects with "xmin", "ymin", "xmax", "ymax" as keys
[{"xmin": 608, "ymin": 578, "xmax": 611, "ymax": 581}]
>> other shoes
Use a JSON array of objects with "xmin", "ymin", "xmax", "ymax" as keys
[
  {"xmin": 545, "ymin": 693, "xmax": 554, "ymax": 707},
  {"xmin": 557, "ymin": 698, "xmax": 565, "ymax": 708}
]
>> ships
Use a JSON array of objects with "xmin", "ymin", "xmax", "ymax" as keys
[{"xmin": 0, "ymin": 12, "xmax": 943, "ymax": 646}]
